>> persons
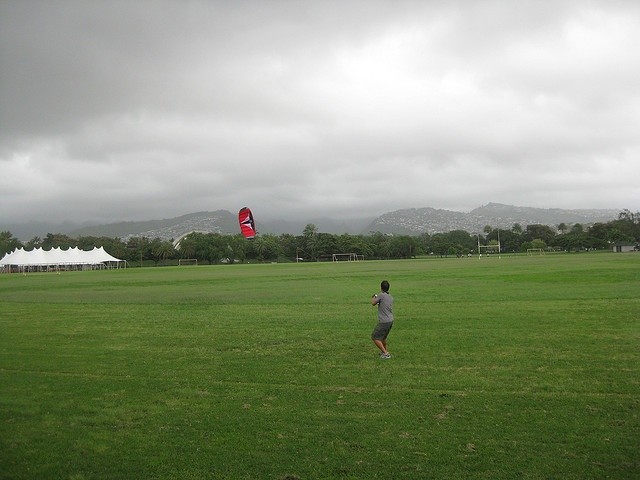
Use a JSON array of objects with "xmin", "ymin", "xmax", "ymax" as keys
[{"xmin": 372, "ymin": 280, "xmax": 395, "ymax": 358}]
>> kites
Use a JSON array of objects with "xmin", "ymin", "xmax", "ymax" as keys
[{"xmin": 239, "ymin": 207, "xmax": 257, "ymax": 240}]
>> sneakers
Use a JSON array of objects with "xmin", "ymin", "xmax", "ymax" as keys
[{"xmin": 379, "ymin": 352, "xmax": 391, "ymax": 358}]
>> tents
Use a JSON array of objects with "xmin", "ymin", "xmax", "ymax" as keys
[{"xmin": 0, "ymin": 244, "xmax": 131, "ymax": 273}]
